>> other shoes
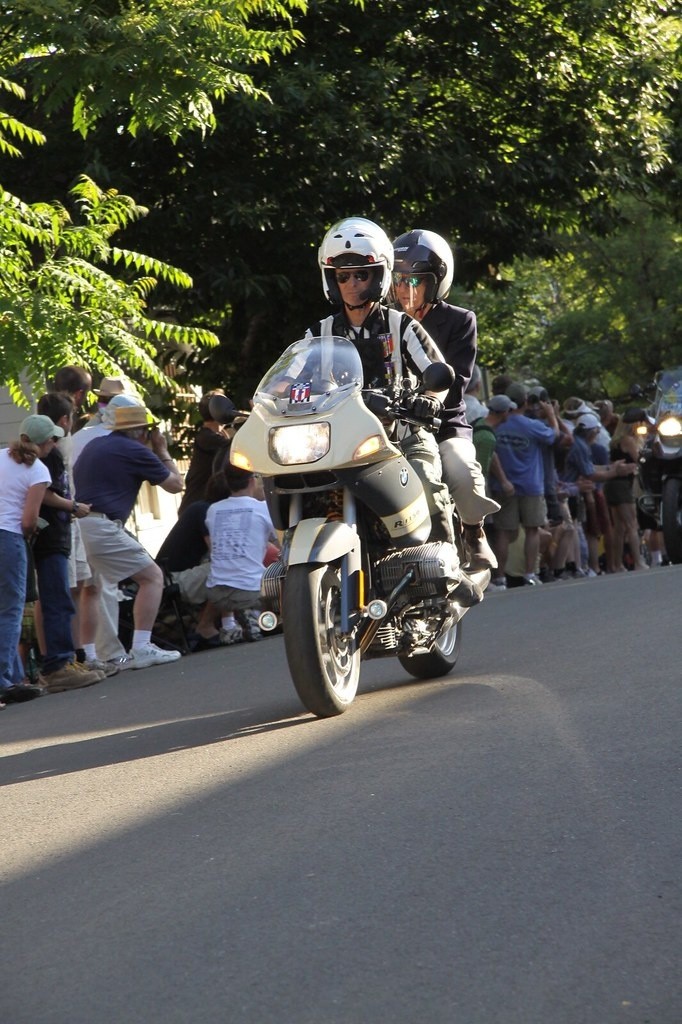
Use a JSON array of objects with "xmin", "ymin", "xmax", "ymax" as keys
[{"xmin": 483, "ymin": 547, "xmax": 669, "ymax": 594}]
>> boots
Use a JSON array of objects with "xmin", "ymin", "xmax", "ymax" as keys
[
  {"xmin": 431, "ymin": 511, "xmax": 485, "ymax": 607},
  {"xmin": 463, "ymin": 519, "xmax": 499, "ymax": 571}
]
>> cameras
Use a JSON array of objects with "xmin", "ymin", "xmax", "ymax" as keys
[{"xmin": 147, "ymin": 430, "xmax": 152, "ymax": 440}]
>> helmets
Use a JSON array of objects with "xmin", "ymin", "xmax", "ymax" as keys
[
  {"xmin": 318, "ymin": 217, "xmax": 395, "ymax": 305},
  {"xmin": 392, "ymin": 229, "xmax": 454, "ymax": 304}
]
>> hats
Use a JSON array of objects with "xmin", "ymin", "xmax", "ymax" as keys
[
  {"xmin": 19, "ymin": 414, "xmax": 65, "ymax": 445},
  {"xmin": 486, "ymin": 394, "xmax": 517, "ymax": 411},
  {"xmin": 104, "ymin": 405, "xmax": 161, "ymax": 433},
  {"xmin": 567, "ymin": 401, "xmax": 591, "ymax": 413},
  {"xmin": 576, "ymin": 414, "xmax": 601, "ymax": 429},
  {"xmin": 92, "ymin": 376, "xmax": 132, "ymax": 397},
  {"xmin": 585, "ymin": 401, "xmax": 600, "ymax": 409},
  {"xmin": 107, "ymin": 395, "xmax": 140, "ymax": 408}
]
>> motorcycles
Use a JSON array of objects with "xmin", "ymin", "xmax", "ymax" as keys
[
  {"xmin": 623, "ymin": 368, "xmax": 682, "ymax": 565},
  {"xmin": 209, "ymin": 337, "xmax": 492, "ymax": 716}
]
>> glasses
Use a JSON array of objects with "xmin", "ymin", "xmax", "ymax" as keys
[
  {"xmin": 50, "ymin": 436, "xmax": 58, "ymax": 443},
  {"xmin": 334, "ymin": 269, "xmax": 380, "ymax": 284},
  {"xmin": 392, "ymin": 274, "xmax": 431, "ymax": 287}
]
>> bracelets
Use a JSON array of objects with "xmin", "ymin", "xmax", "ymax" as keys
[{"xmin": 163, "ymin": 458, "xmax": 172, "ymax": 462}]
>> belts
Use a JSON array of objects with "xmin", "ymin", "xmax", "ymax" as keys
[{"xmin": 87, "ymin": 510, "xmax": 111, "ymax": 521}]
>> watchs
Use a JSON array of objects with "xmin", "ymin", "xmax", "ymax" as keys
[{"xmin": 72, "ymin": 502, "xmax": 79, "ymax": 513}]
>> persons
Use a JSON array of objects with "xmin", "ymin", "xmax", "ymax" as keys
[
  {"xmin": 248, "ymin": 216, "xmax": 502, "ymax": 574},
  {"xmin": 463, "ymin": 362, "xmax": 670, "ymax": 590},
  {"xmin": 0, "ymin": 366, "xmax": 284, "ymax": 709}
]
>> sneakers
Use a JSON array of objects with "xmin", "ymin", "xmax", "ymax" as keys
[{"xmin": 0, "ymin": 609, "xmax": 268, "ymax": 709}]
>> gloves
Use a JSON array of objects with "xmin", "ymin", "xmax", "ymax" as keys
[{"xmin": 401, "ymin": 394, "xmax": 445, "ymax": 429}]
